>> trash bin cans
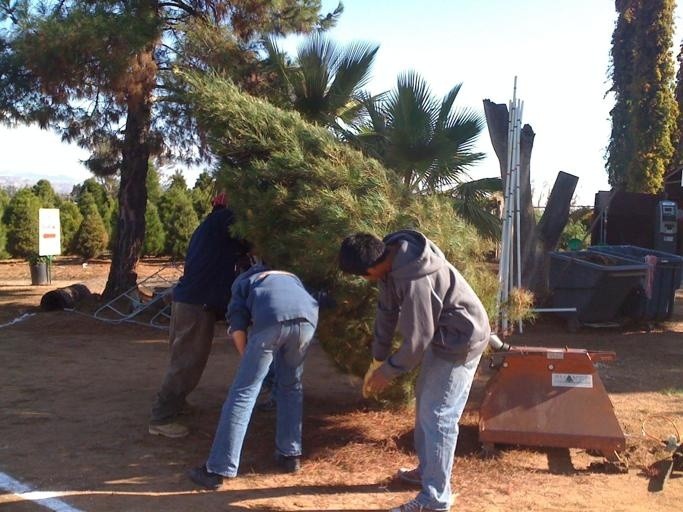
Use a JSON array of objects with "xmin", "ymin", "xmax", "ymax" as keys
[
  {"xmin": 588, "ymin": 245, "xmax": 683, "ymax": 321},
  {"xmin": 549, "ymin": 250, "xmax": 654, "ymax": 331}
]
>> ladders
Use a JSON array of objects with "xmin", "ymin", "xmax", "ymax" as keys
[
  {"xmin": 150, "ymin": 304, "xmax": 172, "ymax": 331},
  {"xmin": 94, "ymin": 256, "xmax": 185, "ymax": 323}
]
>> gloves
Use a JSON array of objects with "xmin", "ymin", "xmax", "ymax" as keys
[{"xmin": 361, "ymin": 357, "xmax": 389, "ymax": 401}]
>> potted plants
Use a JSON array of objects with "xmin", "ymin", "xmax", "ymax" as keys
[{"xmin": 28, "ymin": 254, "xmax": 51, "ymax": 285}]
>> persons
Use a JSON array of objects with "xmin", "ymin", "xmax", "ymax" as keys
[
  {"xmin": 191, "ymin": 264, "xmax": 320, "ymax": 490},
  {"xmin": 149, "ymin": 193, "xmax": 251, "ymax": 438},
  {"xmin": 338, "ymin": 231, "xmax": 491, "ymax": 512}
]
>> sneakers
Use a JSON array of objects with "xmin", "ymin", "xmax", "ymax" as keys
[
  {"xmin": 271, "ymin": 448, "xmax": 301, "ymax": 473},
  {"xmin": 388, "ymin": 467, "xmax": 451, "ymax": 512},
  {"xmin": 256, "ymin": 398, "xmax": 278, "ymax": 411},
  {"xmin": 190, "ymin": 464, "xmax": 220, "ymax": 491},
  {"xmin": 148, "ymin": 402, "xmax": 198, "ymax": 439}
]
